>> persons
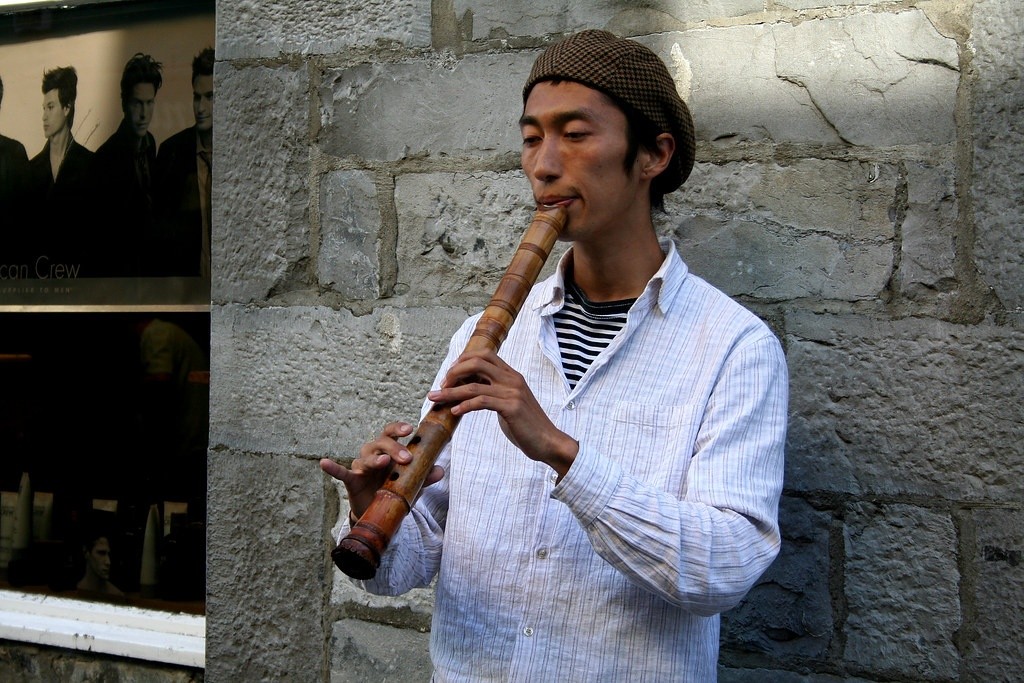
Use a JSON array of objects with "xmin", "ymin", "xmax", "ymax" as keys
[
  {"xmin": 319, "ymin": 31, "xmax": 789, "ymax": 683},
  {"xmin": 68, "ymin": 523, "xmax": 125, "ymax": 604},
  {"xmin": 0, "ymin": 46, "xmax": 215, "ymax": 285}
]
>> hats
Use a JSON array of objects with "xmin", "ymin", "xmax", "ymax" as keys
[{"xmin": 522, "ymin": 29, "xmax": 696, "ymax": 193}]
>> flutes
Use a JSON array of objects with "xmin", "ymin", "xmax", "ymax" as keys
[{"xmin": 330, "ymin": 202, "xmax": 571, "ymax": 581}]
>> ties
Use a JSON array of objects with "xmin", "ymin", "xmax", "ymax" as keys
[{"xmin": 200, "ymin": 149, "xmax": 212, "ymax": 248}]
